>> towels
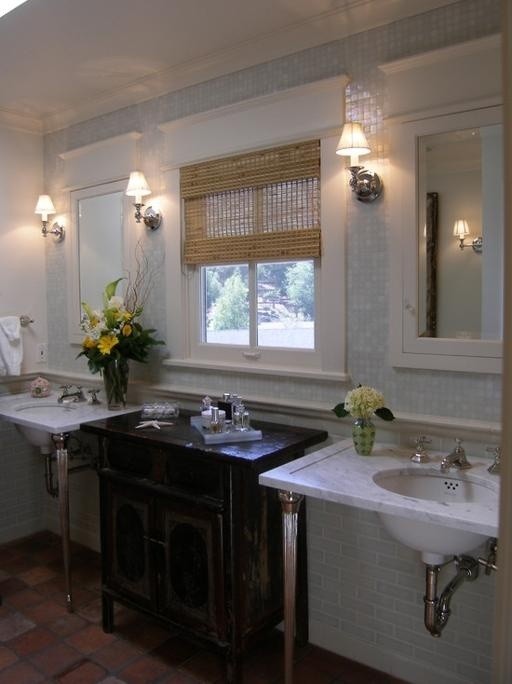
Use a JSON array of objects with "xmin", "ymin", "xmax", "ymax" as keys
[
  {"xmin": 142, "ymin": 400, "xmax": 181, "ymax": 419},
  {"xmin": 0, "ymin": 314, "xmax": 24, "ymax": 378}
]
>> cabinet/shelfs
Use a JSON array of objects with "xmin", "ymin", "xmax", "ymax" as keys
[{"xmin": 79, "ymin": 404, "xmax": 329, "ymax": 683}]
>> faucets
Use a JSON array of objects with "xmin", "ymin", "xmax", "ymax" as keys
[
  {"xmin": 57, "ymin": 385, "xmax": 87, "ymax": 404},
  {"xmin": 439, "ymin": 446, "xmax": 473, "ymax": 474}
]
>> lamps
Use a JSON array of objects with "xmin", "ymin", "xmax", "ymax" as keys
[
  {"xmin": 332, "ymin": 118, "xmax": 383, "ymax": 205},
  {"xmin": 450, "ymin": 216, "xmax": 481, "ymax": 252},
  {"xmin": 123, "ymin": 168, "xmax": 163, "ymax": 227},
  {"xmin": 32, "ymin": 190, "xmax": 69, "ymax": 243}
]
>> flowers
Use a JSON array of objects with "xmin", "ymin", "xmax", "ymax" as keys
[
  {"xmin": 329, "ymin": 381, "xmax": 403, "ymax": 427},
  {"xmin": 74, "ymin": 268, "xmax": 168, "ymax": 405}
]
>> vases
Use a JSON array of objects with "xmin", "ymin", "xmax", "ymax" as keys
[
  {"xmin": 348, "ymin": 415, "xmax": 377, "ymax": 455},
  {"xmin": 104, "ymin": 355, "xmax": 130, "ymax": 414}
]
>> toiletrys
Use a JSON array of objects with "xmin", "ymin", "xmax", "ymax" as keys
[{"xmin": 200, "ymin": 393, "xmax": 249, "ymax": 433}]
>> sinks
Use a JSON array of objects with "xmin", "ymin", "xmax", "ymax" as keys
[
  {"xmin": 371, "ymin": 467, "xmax": 500, "ymax": 557},
  {"xmin": 13, "ymin": 400, "xmax": 78, "ymax": 448}
]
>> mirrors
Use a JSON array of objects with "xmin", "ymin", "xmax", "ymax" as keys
[
  {"xmin": 383, "ymin": 92, "xmax": 503, "ymax": 378},
  {"xmin": 67, "ymin": 177, "xmax": 139, "ymax": 351}
]
